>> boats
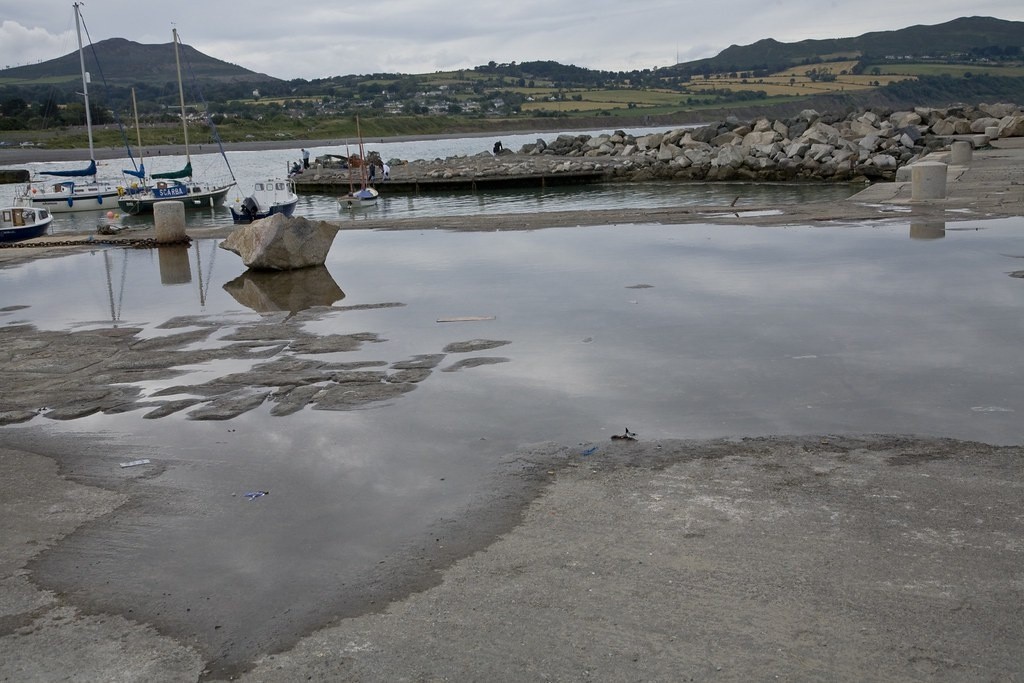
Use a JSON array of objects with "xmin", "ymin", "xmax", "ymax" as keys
[
  {"xmin": 0, "ymin": 183, "xmax": 54, "ymax": 243},
  {"xmin": 223, "ymin": 175, "xmax": 299, "ymax": 222}
]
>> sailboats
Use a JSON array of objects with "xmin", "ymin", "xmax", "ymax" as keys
[
  {"xmin": 116, "ymin": 22, "xmax": 237, "ymax": 216},
  {"xmin": 335, "ymin": 116, "xmax": 380, "ymax": 210},
  {"xmin": 11, "ymin": 1, "xmax": 157, "ymax": 214}
]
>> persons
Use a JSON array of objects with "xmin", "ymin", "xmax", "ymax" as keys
[
  {"xmin": 301, "ymin": 149, "xmax": 310, "ymax": 170},
  {"xmin": 290, "ymin": 162, "xmax": 299, "ymax": 174},
  {"xmin": 494, "ymin": 140, "xmax": 502, "ymax": 155},
  {"xmin": 379, "ymin": 164, "xmax": 390, "ymax": 181},
  {"xmin": 368, "ymin": 161, "xmax": 375, "ymax": 181}
]
